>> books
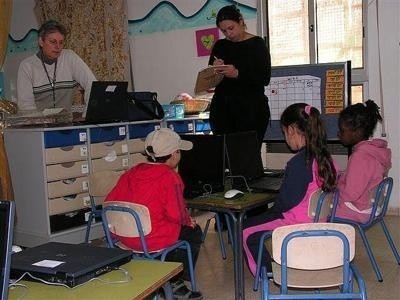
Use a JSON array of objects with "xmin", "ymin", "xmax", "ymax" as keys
[{"xmin": 195, "ymin": 65, "xmax": 228, "ymax": 94}]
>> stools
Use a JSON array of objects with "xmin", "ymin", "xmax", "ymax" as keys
[{"xmin": 183, "ymin": 191, "xmax": 278, "ymax": 300}]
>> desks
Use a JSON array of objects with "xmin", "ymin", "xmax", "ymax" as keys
[{"xmin": 5, "ymin": 260, "xmax": 184, "ymax": 300}]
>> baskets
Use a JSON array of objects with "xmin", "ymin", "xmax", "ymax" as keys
[{"xmin": 170, "ymin": 95, "xmax": 209, "ymax": 112}]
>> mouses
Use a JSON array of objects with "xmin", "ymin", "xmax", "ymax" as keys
[{"xmin": 223, "ymin": 189, "xmax": 245, "ymax": 200}]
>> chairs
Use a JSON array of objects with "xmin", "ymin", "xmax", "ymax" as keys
[
  {"xmin": 85, "ymin": 170, "xmax": 120, "ymax": 249},
  {"xmin": 261, "ymin": 223, "xmax": 368, "ymax": 300},
  {"xmin": 254, "ymin": 186, "xmax": 338, "ymax": 292},
  {"xmin": 327, "ymin": 178, "xmax": 400, "ymax": 282},
  {"xmin": 100, "ymin": 201, "xmax": 196, "ymax": 300}
]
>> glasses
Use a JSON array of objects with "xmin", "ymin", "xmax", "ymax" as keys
[{"xmin": 48, "ymin": 39, "xmax": 66, "ymax": 45}]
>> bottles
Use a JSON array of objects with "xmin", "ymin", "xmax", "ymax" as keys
[{"xmin": 223, "ymin": 169, "xmax": 232, "ymax": 193}]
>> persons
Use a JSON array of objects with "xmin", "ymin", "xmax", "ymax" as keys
[
  {"xmin": 242, "ymin": 102, "xmax": 339, "ymax": 280},
  {"xmin": 17, "ymin": 20, "xmax": 100, "ymax": 109},
  {"xmin": 105, "ymin": 128, "xmax": 204, "ymax": 300},
  {"xmin": 208, "ymin": 5, "xmax": 271, "ymax": 231},
  {"xmin": 334, "ymin": 99, "xmax": 392, "ymax": 222}
]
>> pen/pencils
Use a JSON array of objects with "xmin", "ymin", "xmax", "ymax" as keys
[{"xmin": 213, "ymin": 56, "xmax": 224, "ymax": 66}]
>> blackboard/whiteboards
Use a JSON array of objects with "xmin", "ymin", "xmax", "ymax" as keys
[{"xmin": 263, "ymin": 60, "xmax": 351, "ymax": 143}]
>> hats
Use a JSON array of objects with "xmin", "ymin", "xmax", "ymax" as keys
[{"xmin": 144, "ymin": 129, "xmax": 194, "ymax": 162}]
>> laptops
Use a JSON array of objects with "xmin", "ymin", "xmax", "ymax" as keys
[
  {"xmin": 72, "ymin": 81, "xmax": 128, "ymax": 124},
  {"xmin": 178, "ymin": 135, "xmax": 225, "ymax": 201},
  {"xmin": 225, "ymin": 131, "xmax": 283, "ymax": 192},
  {"xmin": 0, "ymin": 200, "xmax": 16, "ymax": 299},
  {"xmin": 10, "ymin": 242, "xmax": 134, "ymax": 288}
]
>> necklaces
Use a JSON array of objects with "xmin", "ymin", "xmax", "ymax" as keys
[{"xmin": 40, "ymin": 52, "xmax": 57, "ymax": 107}]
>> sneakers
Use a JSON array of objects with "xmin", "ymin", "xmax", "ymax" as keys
[{"xmin": 159, "ymin": 280, "xmax": 203, "ymax": 300}]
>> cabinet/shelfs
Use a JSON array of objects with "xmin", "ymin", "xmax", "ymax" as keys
[{"xmin": 3, "ymin": 116, "xmax": 226, "ymax": 244}]
